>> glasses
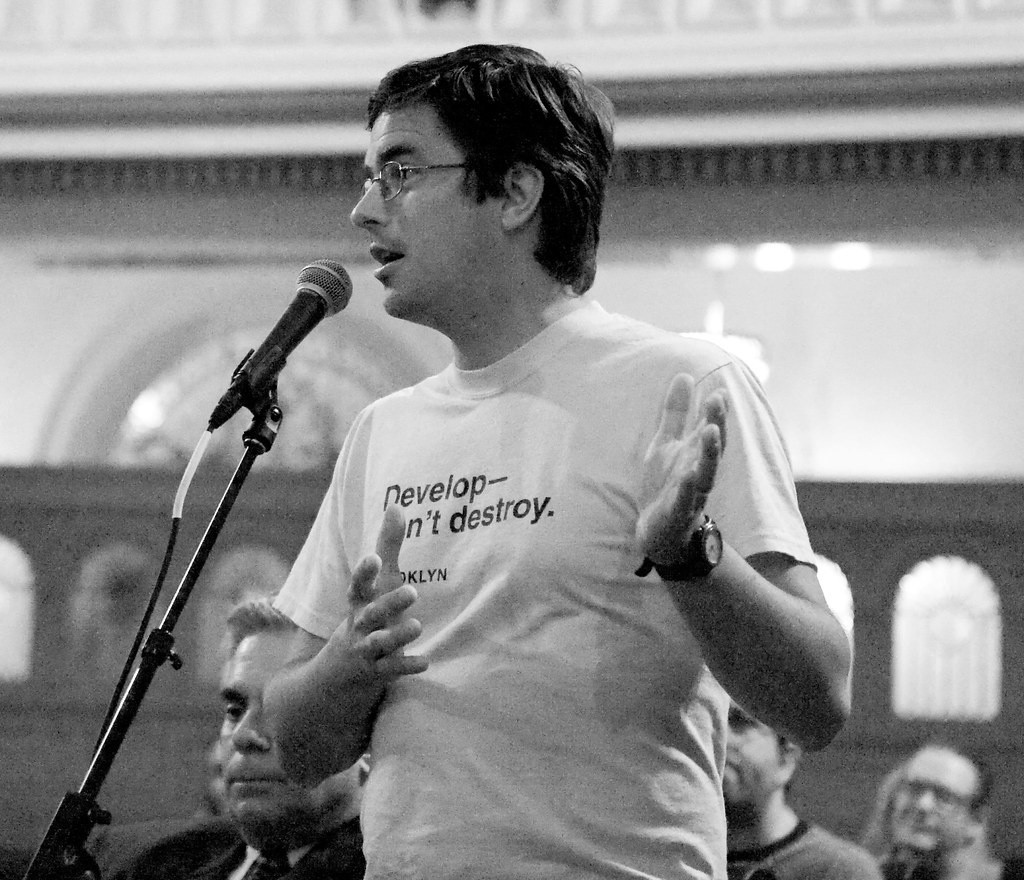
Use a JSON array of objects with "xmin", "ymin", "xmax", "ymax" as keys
[{"xmin": 359, "ymin": 162, "xmax": 489, "ymax": 201}]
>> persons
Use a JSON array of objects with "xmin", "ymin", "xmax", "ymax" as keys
[
  {"xmin": 880, "ymin": 738, "xmax": 1022, "ymax": 880},
  {"xmin": 262, "ymin": 46, "xmax": 858, "ymax": 879},
  {"xmin": 108, "ymin": 596, "xmax": 364, "ymax": 879},
  {"xmin": 723, "ymin": 688, "xmax": 878, "ymax": 880}
]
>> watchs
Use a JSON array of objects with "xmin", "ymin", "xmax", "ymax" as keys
[{"xmin": 634, "ymin": 516, "xmax": 724, "ymax": 584}]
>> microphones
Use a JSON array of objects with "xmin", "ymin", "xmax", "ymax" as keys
[{"xmin": 208, "ymin": 260, "xmax": 352, "ymax": 429}]
